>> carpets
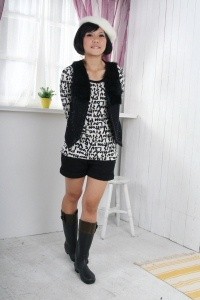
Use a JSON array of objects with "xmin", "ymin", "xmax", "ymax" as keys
[{"xmin": 132, "ymin": 253, "xmax": 200, "ymax": 300}]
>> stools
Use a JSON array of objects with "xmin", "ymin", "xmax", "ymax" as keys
[{"xmin": 100, "ymin": 176, "xmax": 135, "ymax": 239}]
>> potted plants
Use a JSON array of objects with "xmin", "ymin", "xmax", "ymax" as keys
[{"xmin": 38, "ymin": 87, "xmax": 56, "ymax": 109}]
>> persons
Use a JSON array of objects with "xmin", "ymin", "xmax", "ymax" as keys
[{"xmin": 59, "ymin": 16, "xmax": 126, "ymax": 284}]
